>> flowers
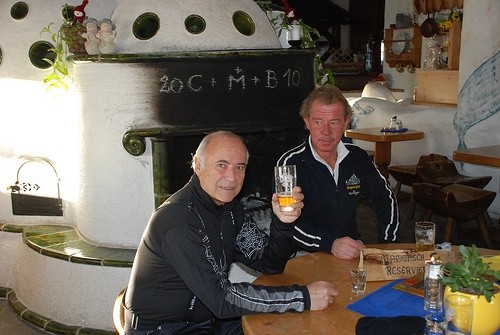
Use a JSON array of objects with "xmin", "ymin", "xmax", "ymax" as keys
[{"xmin": 440, "ymin": 6, "xmax": 463, "ymax": 28}]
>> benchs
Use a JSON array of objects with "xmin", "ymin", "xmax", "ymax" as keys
[{"xmin": 0, "ymin": 222, "xmax": 137, "ymax": 335}]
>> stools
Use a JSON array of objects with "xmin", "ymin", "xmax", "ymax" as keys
[{"xmin": 387, "ymin": 153, "xmax": 496, "ymax": 251}]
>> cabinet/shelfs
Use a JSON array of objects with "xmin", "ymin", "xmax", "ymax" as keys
[{"xmin": 384, "ymin": 26, "xmax": 422, "ymax": 67}]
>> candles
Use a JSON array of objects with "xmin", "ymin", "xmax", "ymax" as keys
[{"xmin": 289, "ymin": 25, "xmax": 300, "ymax": 41}]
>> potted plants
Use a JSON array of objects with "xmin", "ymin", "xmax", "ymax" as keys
[
  {"xmin": 442, "ymin": 244, "xmax": 500, "ymax": 335},
  {"xmin": 42, "ymin": 12, "xmax": 95, "ymax": 91},
  {"xmin": 268, "ymin": 15, "xmax": 315, "ymax": 48}
]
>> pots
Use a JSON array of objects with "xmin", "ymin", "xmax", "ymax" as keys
[{"xmin": 421, "ymin": 13, "xmax": 438, "ymax": 38}]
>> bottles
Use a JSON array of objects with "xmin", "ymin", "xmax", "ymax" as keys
[
  {"xmin": 424, "ymin": 314, "xmax": 447, "ymax": 335},
  {"xmin": 423, "ymin": 258, "xmax": 443, "ymax": 313},
  {"xmin": 395, "ymin": 120, "xmax": 402, "ymax": 131},
  {"xmin": 389, "ymin": 116, "xmax": 396, "ymax": 130}
]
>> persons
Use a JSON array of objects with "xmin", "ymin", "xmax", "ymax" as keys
[
  {"xmin": 121, "ymin": 132, "xmax": 338, "ymax": 335},
  {"xmin": 271, "ymin": 83, "xmax": 400, "ymax": 261}
]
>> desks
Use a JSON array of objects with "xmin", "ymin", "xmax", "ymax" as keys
[
  {"xmin": 453, "ymin": 145, "xmax": 500, "ymax": 168},
  {"xmin": 345, "ymin": 128, "xmax": 424, "ymax": 185},
  {"xmin": 241, "ymin": 242, "xmax": 500, "ymax": 334}
]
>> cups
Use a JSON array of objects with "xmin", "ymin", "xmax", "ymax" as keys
[
  {"xmin": 442, "ymin": 293, "xmax": 474, "ymax": 335},
  {"xmin": 414, "ymin": 221, "xmax": 436, "ymax": 252},
  {"xmin": 350, "ymin": 265, "xmax": 368, "ymax": 296},
  {"xmin": 274, "ymin": 166, "xmax": 298, "ymax": 212}
]
